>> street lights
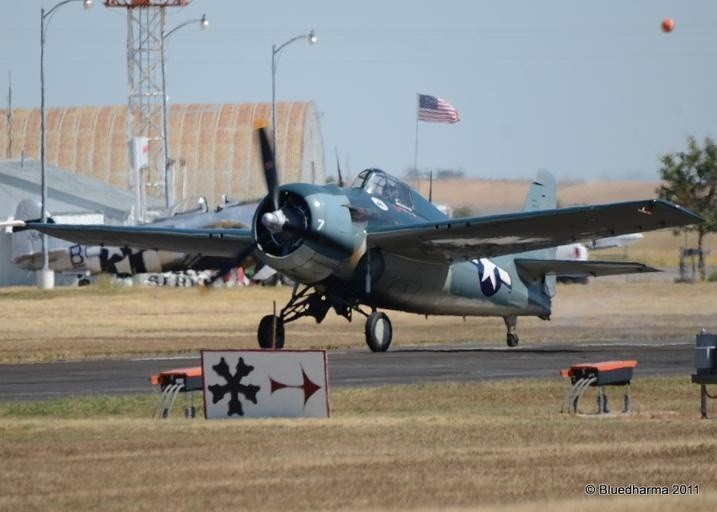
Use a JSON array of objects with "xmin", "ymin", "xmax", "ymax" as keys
[
  {"xmin": 271, "ymin": 26, "xmax": 326, "ymax": 192},
  {"xmin": 36, "ymin": 0, "xmax": 98, "ymax": 292},
  {"xmin": 161, "ymin": 13, "xmax": 211, "ymax": 213}
]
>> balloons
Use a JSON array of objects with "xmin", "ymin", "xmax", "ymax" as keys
[{"xmin": 660, "ymin": 15, "xmax": 677, "ymax": 32}]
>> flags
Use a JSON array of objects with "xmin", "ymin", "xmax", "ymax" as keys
[{"xmin": 417, "ymin": 91, "xmax": 460, "ymax": 124}]
